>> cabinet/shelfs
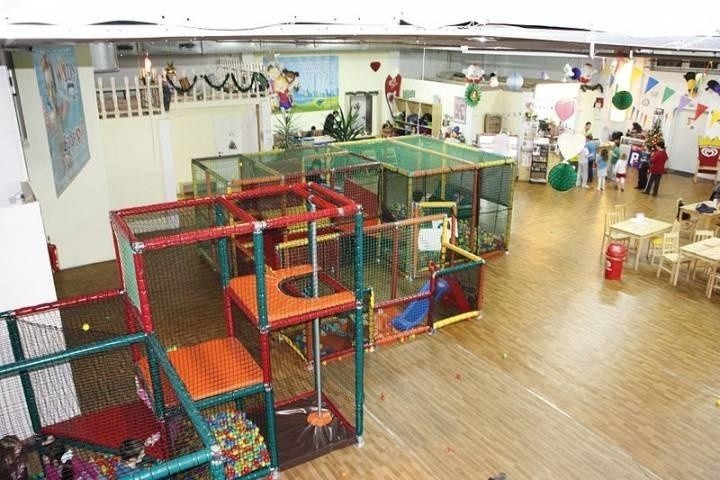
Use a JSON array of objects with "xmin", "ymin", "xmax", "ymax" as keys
[{"xmin": 392, "ymin": 96, "xmax": 442, "ymax": 138}]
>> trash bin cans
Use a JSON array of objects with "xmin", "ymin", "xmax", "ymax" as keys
[{"xmin": 605, "ymin": 242, "xmax": 626, "ymax": 280}]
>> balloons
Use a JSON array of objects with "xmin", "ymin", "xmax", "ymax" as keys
[
  {"xmin": 555, "ymin": 100, "xmax": 574, "ymax": 121},
  {"xmin": 557, "ymin": 131, "xmax": 585, "ymax": 159}
]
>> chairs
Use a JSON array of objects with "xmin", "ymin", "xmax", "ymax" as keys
[{"xmin": 600, "ymin": 197, "xmax": 720, "ymax": 299}]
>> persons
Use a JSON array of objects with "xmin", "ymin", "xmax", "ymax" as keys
[
  {"xmin": 322, "ymin": 110, "xmax": 339, "ymax": 135},
  {"xmin": 575, "ymin": 122, "xmax": 627, "ymax": 193},
  {"xmin": 0, "ymin": 432, "xmax": 97, "ymax": 480},
  {"xmin": 305, "ymin": 158, "xmax": 322, "ymax": 184},
  {"xmin": 114, "ymin": 438, "xmax": 146, "ymax": 480},
  {"xmin": 634, "ymin": 141, "xmax": 668, "ymax": 196},
  {"xmin": 412, "ymin": 189, "xmax": 451, "ymax": 216},
  {"xmin": 305, "ymin": 126, "xmax": 316, "ymax": 137}
]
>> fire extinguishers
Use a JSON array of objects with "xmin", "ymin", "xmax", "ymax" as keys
[{"xmin": 47, "ymin": 236, "xmax": 60, "ymax": 273}]
